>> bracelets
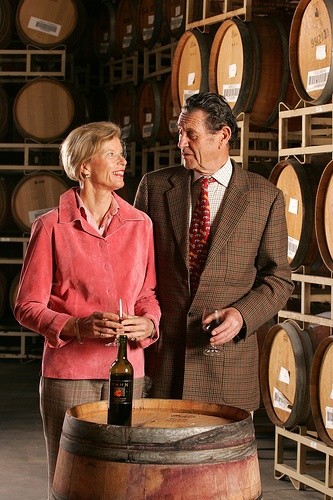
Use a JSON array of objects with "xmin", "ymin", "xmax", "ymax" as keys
[{"xmin": 74, "ymin": 318, "xmax": 84, "ymax": 344}]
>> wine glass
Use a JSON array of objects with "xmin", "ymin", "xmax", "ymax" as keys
[
  {"xmin": 105, "ymin": 298, "xmax": 128, "ymax": 346},
  {"xmin": 202, "ymin": 308, "xmax": 224, "ymax": 356}
]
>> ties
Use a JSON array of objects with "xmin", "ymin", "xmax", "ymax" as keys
[{"xmin": 188, "ymin": 176, "xmax": 217, "ymax": 291}]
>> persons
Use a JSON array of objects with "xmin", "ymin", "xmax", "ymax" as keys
[
  {"xmin": 133, "ymin": 93, "xmax": 292, "ymax": 413},
  {"xmin": 12, "ymin": 121, "xmax": 161, "ymax": 500}
]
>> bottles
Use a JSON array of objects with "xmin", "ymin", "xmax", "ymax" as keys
[{"xmin": 107, "ymin": 335, "xmax": 134, "ymax": 426}]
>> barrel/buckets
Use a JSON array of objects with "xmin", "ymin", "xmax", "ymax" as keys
[
  {"xmin": 315, "ymin": 159, "xmax": 333, "ymax": 272},
  {"xmin": 0, "ymin": 0, "xmax": 21, "ymax": 324},
  {"xmin": 11, "ymin": 172, "xmax": 70, "ymax": 232},
  {"xmin": 51, "ymin": 399, "xmax": 263, "ymax": 500},
  {"xmin": 309, "ymin": 335, "xmax": 333, "ymax": 449},
  {"xmin": 260, "ymin": 313, "xmax": 333, "ymax": 430},
  {"xmin": 16, "ymin": 0, "xmax": 78, "ymax": 48},
  {"xmin": 171, "ymin": 30, "xmax": 218, "ymax": 115},
  {"xmin": 268, "ymin": 160, "xmax": 331, "ymax": 275},
  {"xmin": 13, "ymin": 79, "xmax": 75, "ymax": 143},
  {"xmin": 92, "ymin": 0, "xmax": 203, "ymax": 144},
  {"xmin": 289, "ymin": 0, "xmax": 333, "ymax": 105},
  {"xmin": 208, "ymin": 19, "xmax": 332, "ymax": 131}
]
